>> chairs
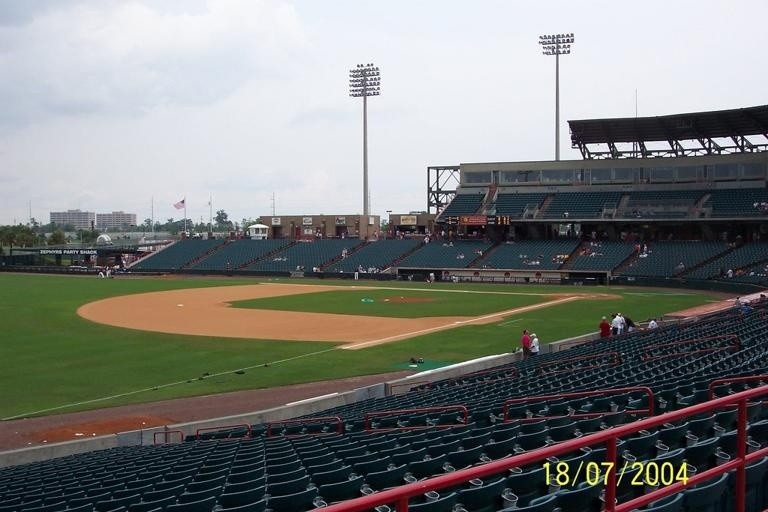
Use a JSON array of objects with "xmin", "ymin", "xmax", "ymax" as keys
[{"xmin": 318, "ymin": 348, "xmax": 547, "ymax": 510}]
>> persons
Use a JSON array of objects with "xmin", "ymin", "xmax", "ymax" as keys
[
  {"xmin": 94, "ymin": 265, "xmax": 114, "ymax": 279},
  {"xmin": 521, "ymin": 329, "xmax": 531, "ymax": 359},
  {"xmin": 273, "ymin": 225, "xmax": 768, "ymax": 285},
  {"xmin": 598, "ymin": 291, "xmax": 767, "ymax": 338},
  {"xmin": 528, "ymin": 333, "xmax": 539, "ymax": 356}
]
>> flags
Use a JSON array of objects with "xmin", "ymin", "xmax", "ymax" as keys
[{"xmin": 173, "ymin": 199, "xmax": 184, "ymax": 210}]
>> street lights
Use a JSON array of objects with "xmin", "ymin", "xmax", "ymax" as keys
[
  {"xmin": 540, "ymin": 33, "xmax": 574, "ymax": 161},
  {"xmin": 348, "ymin": 62, "xmax": 380, "ymax": 237}
]
